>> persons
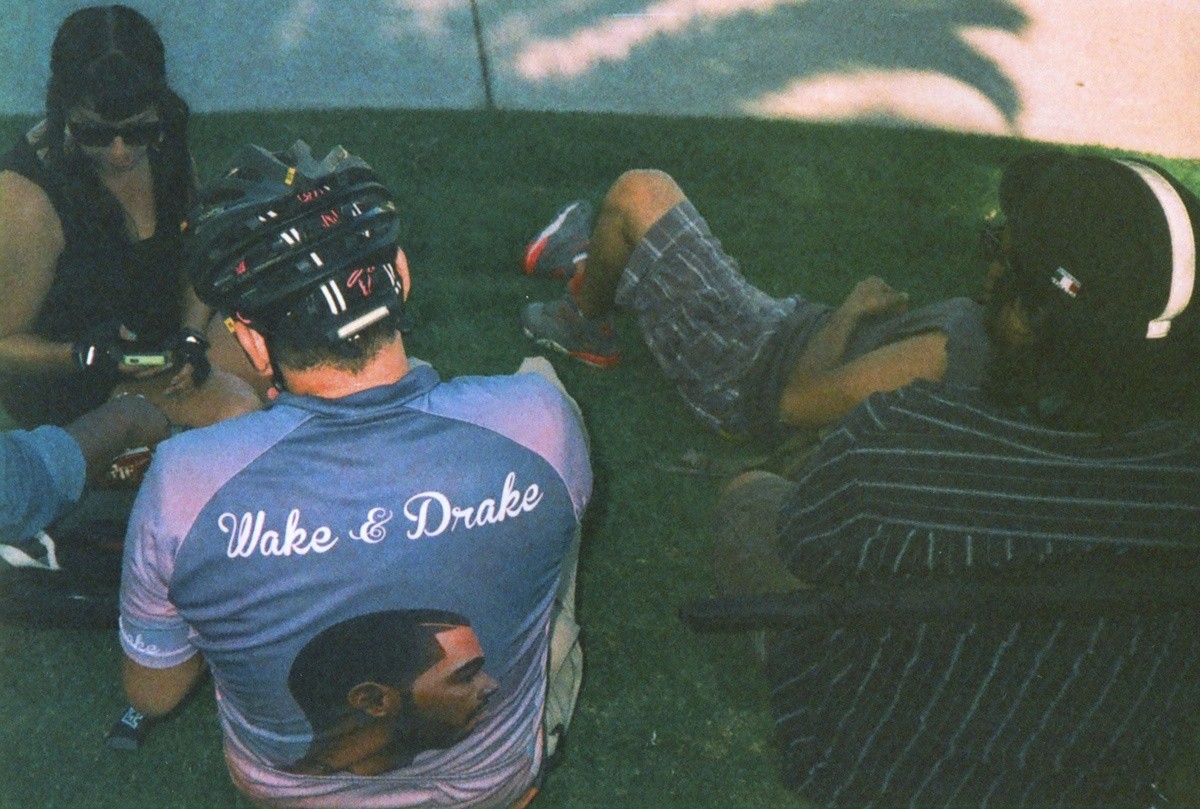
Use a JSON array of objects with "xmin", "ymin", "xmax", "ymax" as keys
[
  {"xmin": 2, "ymin": 7, "xmax": 272, "ymax": 430},
  {"xmin": 120, "ymin": 139, "xmax": 599, "ymax": 809},
  {"xmin": 527, "ymin": 147, "xmax": 1200, "ymax": 443},
  {"xmin": 290, "ymin": 608, "xmax": 500, "ymax": 777},
  {"xmin": 2, "ymin": 392, "xmax": 172, "ymax": 547}
]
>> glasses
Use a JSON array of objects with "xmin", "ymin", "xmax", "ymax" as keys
[
  {"xmin": 67, "ymin": 112, "xmax": 161, "ymax": 148},
  {"xmin": 980, "ymin": 225, "xmax": 1013, "ymax": 272}
]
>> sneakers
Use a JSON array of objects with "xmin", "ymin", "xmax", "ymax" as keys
[
  {"xmin": 518, "ymin": 299, "xmax": 624, "ymax": 368},
  {"xmin": 523, "ymin": 198, "xmax": 595, "ymax": 281}
]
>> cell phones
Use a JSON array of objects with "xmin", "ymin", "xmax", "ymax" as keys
[{"xmin": 122, "ymin": 353, "xmax": 168, "ymax": 368}]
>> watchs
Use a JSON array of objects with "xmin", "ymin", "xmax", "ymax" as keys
[{"xmin": 181, "ymin": 328, "xmax": 213, "ymax": 351}]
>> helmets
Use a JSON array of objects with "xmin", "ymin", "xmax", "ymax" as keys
[
  {"xmin": 188, "ymin": 140, "xmax": 404, "ymax": 324},
  {"xmin": 998, "ymin": 148, "xmax": 1200, "ymax": 343}
]
